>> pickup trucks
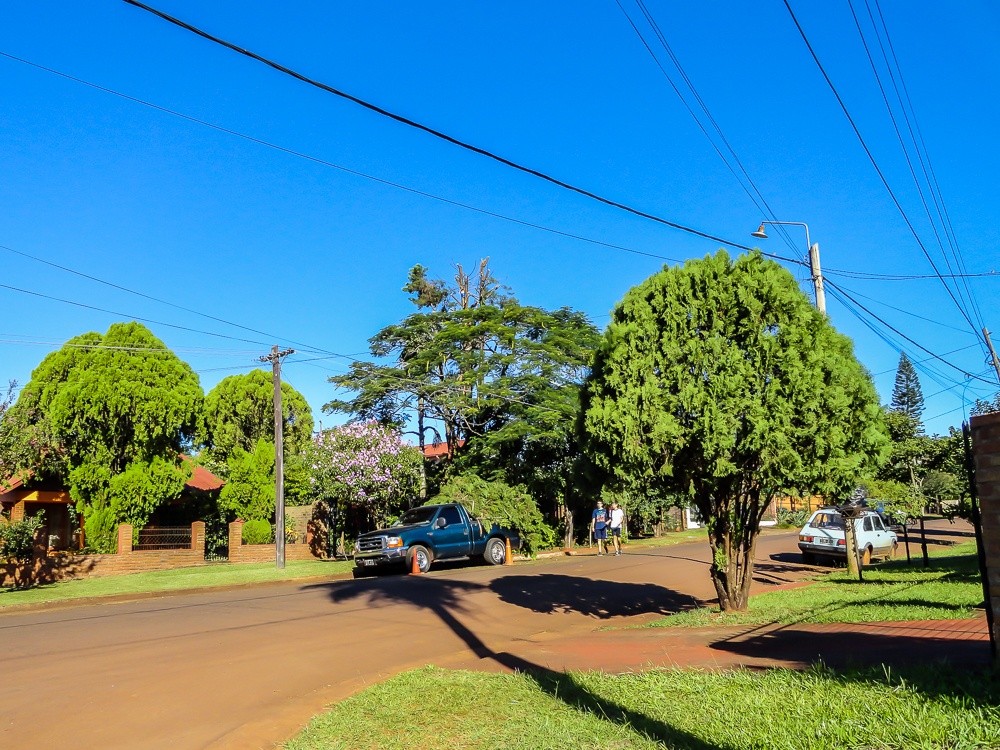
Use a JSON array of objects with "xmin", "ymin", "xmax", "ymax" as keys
[{"xmin": 352, "ymin": 503, "xmax": 520, "ymax": 575}]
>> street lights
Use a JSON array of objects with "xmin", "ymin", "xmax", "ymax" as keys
[{"xmin": 751, "ymin": 221, "xmax": 862, "ymax": 578}]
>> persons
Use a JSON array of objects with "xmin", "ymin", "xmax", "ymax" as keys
[
  {"xmin": 605, "ymin": 501, "xmax": 624, "ymax": 556},
  {"xmin": 591, "ymin": 500, "xmax": 609, "ymax": 557}
]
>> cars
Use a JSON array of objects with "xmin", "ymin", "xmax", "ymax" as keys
[{"xmin": 797, "ymin": 504, "xmax": 900, "ymax": 567}]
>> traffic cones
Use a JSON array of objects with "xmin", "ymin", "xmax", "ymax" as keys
[
  {"xmin": 502, "ymin": 537, "xmax": 518, "ymax": 566},
  {"xmin": 408, "ymin": 548, "xmax": 425, "ymax": 576}
]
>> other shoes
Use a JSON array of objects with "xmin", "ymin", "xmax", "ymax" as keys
[
  {"xmin": 598, "ymin": 552, "xmax": 603, "ymax": 556},
  {"xmin": 606, "ymin": 549, "xmax": 609, "ymax": 554},
  {"xmin": 619, "ymin": 548, "xmax": 623, "ymax": 554},
  {"xmin": 615, "ymin": 550, "xmax": 619, "ymax": 555}
]
